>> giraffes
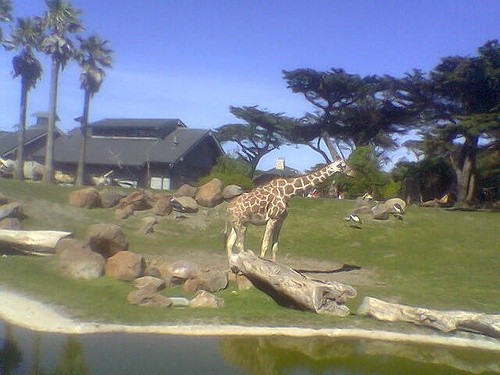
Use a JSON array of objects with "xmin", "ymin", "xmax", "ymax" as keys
[{"xmin": 224, "ymin": 155, "xmax": 356, "ymax": 262}]
